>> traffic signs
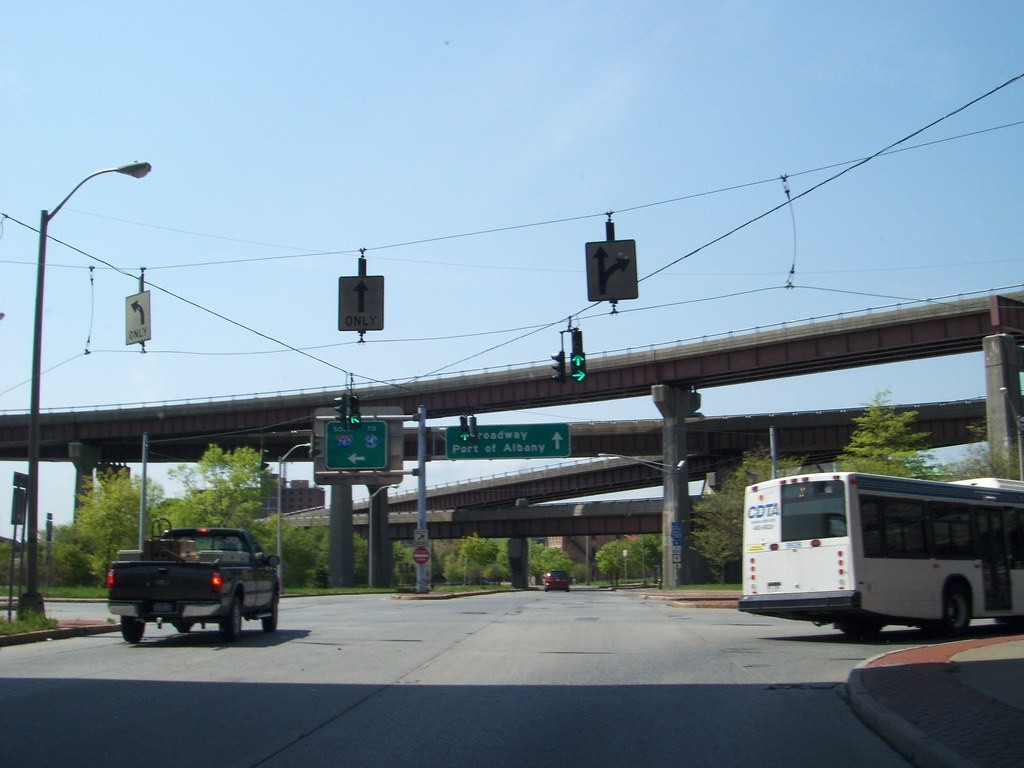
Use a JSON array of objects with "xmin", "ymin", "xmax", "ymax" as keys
[
  {"xmin": 445, "ymin": 424, "xmax": 572, "ymax": 460},
  {"xmin": 325, "ymin": 420, "xmax": 389, "ymax": 470}
]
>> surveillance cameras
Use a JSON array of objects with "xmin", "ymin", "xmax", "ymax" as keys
[{"xmin": 677, "ymin": 460, "xmax": 687, "ymax": 471}]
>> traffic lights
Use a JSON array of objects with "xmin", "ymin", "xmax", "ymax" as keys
[
  {"xmin": 349, "ymin": 396, "xmax": 361, "ymax": 430},
  {"xmin": 551, "ymin": 351, "xmax": 565, "ymax": 382},
  {"xmin": 334, "ymin": 393, "xmax": 346, "ymax": 431},
  {"xmin": 459, "ymin": 415, "xmax": 469, "ymax": 442},
  {"xmin": 570, "ymin": 352, "xmax": 587, "ymax": 382},
  {"xmin": 310, "ymin": 434, "xmax": 322, "ymax": 459}
]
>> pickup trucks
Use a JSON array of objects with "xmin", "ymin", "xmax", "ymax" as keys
[{"xmin": 107, "ymin": 527, "xmax": 280, "ymax": 643}]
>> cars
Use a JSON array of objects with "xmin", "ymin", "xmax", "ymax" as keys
[{"xmin": 543, "ymin": 570, "xmax": 570, "ymax": 592}]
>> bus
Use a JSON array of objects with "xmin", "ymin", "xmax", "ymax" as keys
[{"xmin": 737, "ymin": 471, "xmax": 1024, "ymax": 642}]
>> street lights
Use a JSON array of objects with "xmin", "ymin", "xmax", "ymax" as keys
[
  {"xmin": 598, "ymin": 453, "xmax": 685, "ymax": 589},
  {"xmin": 1000, "ymin": 386, "xmax": 1024, "ymax": 480},
  {"xmin": 369, "ymin": 484, "xmax": 400, "ymax": 588},
  {"xmin": 17, "ymin": 162, "xmax": 152, "ymax": 621}
]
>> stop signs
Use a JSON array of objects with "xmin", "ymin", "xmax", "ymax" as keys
[{"xmin": 413, "ymin": 546, "xmax": 430, "ymax": 564}]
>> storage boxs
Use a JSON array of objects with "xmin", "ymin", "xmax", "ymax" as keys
[{"xmin": 117, "ymin": 538, "xmax": 251, "ymax": 560}]
